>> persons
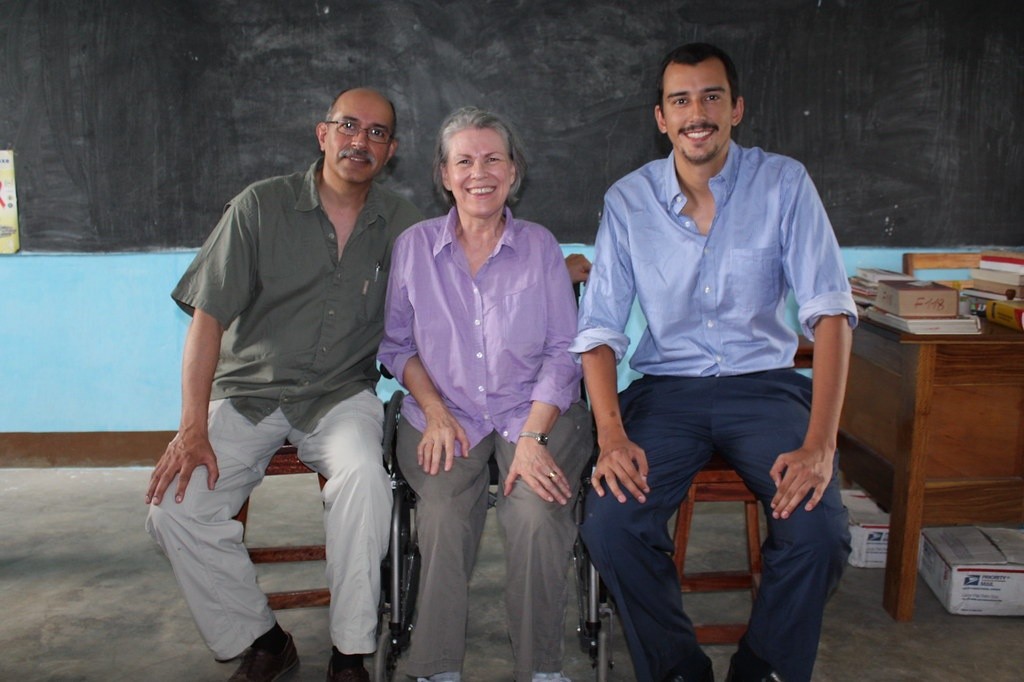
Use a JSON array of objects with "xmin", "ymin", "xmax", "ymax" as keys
[
  {"xmin": 147, "ymin": 88, "xmax": 592, "ymax": 682},
  {"xmin": 376, "ymin": 106, "xmax": 594, "ymax": 682},
  {"xmin": 568, "ymin": 41, "xmax": 858, "ymax": 682}
]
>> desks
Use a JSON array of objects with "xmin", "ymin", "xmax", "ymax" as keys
[{"xmin": 836, "ymin": 318, "xmax": 1024, "ymax": 620}]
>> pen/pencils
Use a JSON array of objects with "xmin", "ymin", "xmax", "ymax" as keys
[{"xmin": 373, "ymin": 262, "xmax": 382, "ymax": 282}]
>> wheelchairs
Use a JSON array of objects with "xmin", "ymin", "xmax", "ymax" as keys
[{"xmin": 373, "ymin": 276, "xmax": 620, "ymax": 682}]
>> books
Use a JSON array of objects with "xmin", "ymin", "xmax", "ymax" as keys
[{"xmin": 850, "ymin": 248, "xmax": 1024, "ymax": 335}]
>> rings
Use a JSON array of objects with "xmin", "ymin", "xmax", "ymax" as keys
[{"xmin": 549, "ymin": 472, "xmax": 556, "ymax": 478}]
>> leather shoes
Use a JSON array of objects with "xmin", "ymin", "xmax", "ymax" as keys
[
  {"xmin": 225, "ymin": 631, "xmax": 300, "ymax": 682},
  {"xmin": 327, "ymin": 656, "xmax": 370, "ymax": 682}
]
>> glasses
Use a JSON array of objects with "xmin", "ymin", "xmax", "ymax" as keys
[{"xmin": 325, "ymin": 121, "xmax": 393, "ymax": 144}]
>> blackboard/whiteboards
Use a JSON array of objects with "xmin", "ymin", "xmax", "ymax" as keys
[{"xmin": 0, "ymin": 0, "xmax": 1024, "ymax": 256}]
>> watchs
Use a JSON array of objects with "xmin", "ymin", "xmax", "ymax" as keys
[{"xmin": 519, "ymin": 432, "xmax": 548, "ymax": 445}]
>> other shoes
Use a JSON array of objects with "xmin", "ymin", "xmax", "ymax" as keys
[
  {"xmin": 660, "ymin": 655, "xmax": 715, "ymax": 682},
  {"xmin": 725, "ymin": 653, "xmax": 787, "ymax": 682}
]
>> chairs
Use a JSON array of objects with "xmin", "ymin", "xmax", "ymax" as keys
[{"xmin": 903, "ymin": 252, "xmax": 982, "ymax": 288}]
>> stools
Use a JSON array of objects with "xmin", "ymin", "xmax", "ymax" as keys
[
  {"xmin": 227, "ymin": 445, "xmax": 332, "ymax": 611},
  {"xmin": 665, "ymin": 465, "xmax": 764, "ymax": 646}
]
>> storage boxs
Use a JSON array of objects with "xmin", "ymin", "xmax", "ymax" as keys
[
  {"xmin": 919, "ymin": 527, "xmax": 1024, "ymax": 620},
  {"xmin": 838, "ymin": 489, "xmax": 890, "ymax": 568}
]
